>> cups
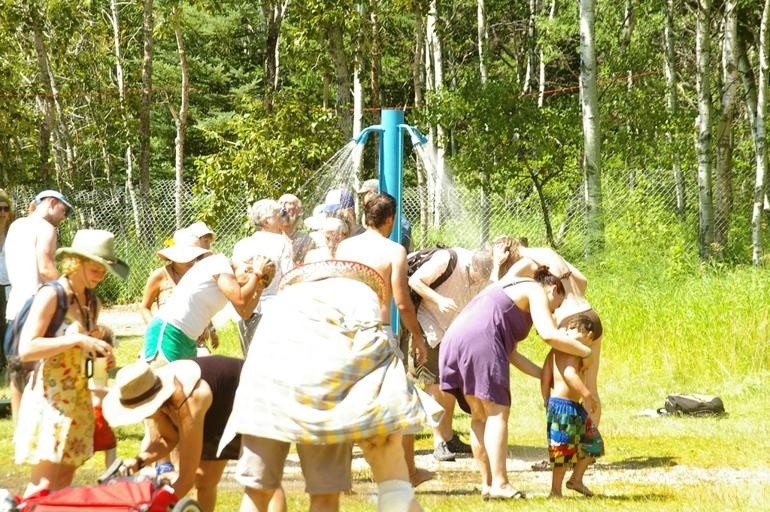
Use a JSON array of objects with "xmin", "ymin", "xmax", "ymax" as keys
[{"xmin": 84, "ymin": 351, "xmax": 109, "ymax": 392}]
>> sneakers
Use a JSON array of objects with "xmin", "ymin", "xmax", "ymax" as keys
[
  {"xmin": 433, "ymin": 441, "xmax": 457, "ymax": 461},
  {"xmin": 532, "ymin": 460, "xmax": 552, "ymax": 470},
  {"xmin": 445, "ymin": 434, "xmax": 471, "ymax": 454}
]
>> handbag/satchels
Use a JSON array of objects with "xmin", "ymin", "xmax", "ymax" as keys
[{"xmin": 406, "ymin": 249, "xmax": 432, "ymax": 297}]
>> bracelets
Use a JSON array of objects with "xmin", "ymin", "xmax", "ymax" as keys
[{"xmin": 134, "ymin": 455, "xmax": 144, "ymax": 471}]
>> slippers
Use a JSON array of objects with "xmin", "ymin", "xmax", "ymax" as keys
[{"xmin": 490, "ymin": 491, "xmax": 524, "ymax": 500}]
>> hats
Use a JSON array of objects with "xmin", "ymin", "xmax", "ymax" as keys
[
  {"xmin": 317, "ymin": 189, "xmax": 356, "ymax": 212},
  {"xmin": 357, "ymin": 179, "xmax": 379, "ymax": 195},
  {"xmin": 185, "ymin": 221, "xmax": 217, "ymax": 243},
  {"xmin": 53, "ymin": 229, "xmax": 131, "ymax": 281},
  {"xmin": 38, "ymin": 189, "xmax": 75, "ymax": 216},
  {"xmin": 100, "ymin": 359, "xmax": 177, "ymax": 427},
  {"xmin": 304, "ymin": 216, "xmax": 350, "ymax": 234},
  {"xmin": 157, "ymin": 234, "xmax": 213, "ymax": 265}
]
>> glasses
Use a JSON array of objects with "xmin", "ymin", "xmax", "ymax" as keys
[{"xmin": 0, "ymin": 206, "xmax": 9, "ymax": 212}]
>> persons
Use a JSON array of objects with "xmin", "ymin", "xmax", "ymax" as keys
[
  {"xmin": 442, "ymin": 264, "xmax": 593, "ymax": 499},
  {"xmin": 232, "ymin": 200, "xmax": 295, "ymax": 358},
  {"xmin": 192, "ymin": 221, "xmax": 216, "ymax": 351},
  {"xmin": 357, "ymin": 435, "xmax": 424, "ymax": 512},
  {"xmin": 322, "ymin": 188, "xmax": 361, "ymax": 234},
  {"xmin": 101, "ymin": 352, "xmax": 286, "ymax": 510},
  {"xmin": 14, "ymin": 230, "xmax": 130, "ymax": 498},
  {"xmin": 497, "ymin": 235, "xmax": 601, "ymax": 467},
  {"xmin": 279, "ymin": 191, "xmax": 315, "ymax": 262},
  {"xmin": 142, "ymin": 228, "xmax": 208, "ymax": 328},
  {"xmin": 304, "ymin": 219, "xmax": 348, "ymax": 264},
  {"xmin": 0, "ymin": 191, "xmax": 82, "ymax": 410},
  {"xmin": 340, "ymin": 195, "xmax": 435, "ymax": 485},
  {"xmin": 0, "ymin": 187, "xmax": 14, "ymax": 419},
  {"xmin": 357, "ymin": 180, "xmax": 413, "ymax": 252},
  {"xmin": 142, "ymin": 255, "xmax": 274, "ymax": 470},
  {"xmin": 540, "ymin": 314, "xmax": 604, "ymax": 501},
  {"xmin": 233, "ymin": 260, "xmax": 387, "ymax": 511},
  {"xmin": 410, "ymin": 246, "xmax": 497, "ymax": 461}
]
